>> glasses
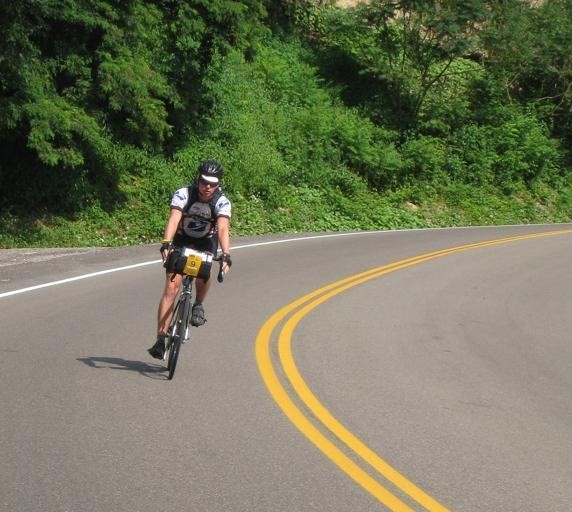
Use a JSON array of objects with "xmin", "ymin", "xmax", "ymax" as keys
[{"xmin": 199, "ymin": 179, "xmax": 219, "ymax": 188}]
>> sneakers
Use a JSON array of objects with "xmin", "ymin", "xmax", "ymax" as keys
[
  {"xmin": 191, "ymin": 301, "xmax": 205, "ymax": 324},
  {"xmin": 151, "ymin": 337, "xmax": 166, "ymax": 358}
]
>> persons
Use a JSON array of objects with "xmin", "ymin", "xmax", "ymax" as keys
[{"xmin": 149, "ymin": 161, "xmax": 232, "ymax": 358}]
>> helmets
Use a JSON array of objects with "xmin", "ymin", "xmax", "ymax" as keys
[{"xmin": 198, "ymin": 159, "xmax": 224, "ymax": 178}]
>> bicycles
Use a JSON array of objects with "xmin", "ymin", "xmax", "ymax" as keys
[{"xmin": 164, "ymin": 245, "xmax": 220, "ymax": 378}]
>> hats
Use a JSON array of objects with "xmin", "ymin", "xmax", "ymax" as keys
[{"xmin": 200, "ymin": 174, "xmax": 220, "ymax": 183}]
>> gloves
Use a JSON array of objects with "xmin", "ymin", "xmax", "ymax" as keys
[
  {"xmin": 221, "ymin": 252, "xmax": 232, "ymax": 267},
  {"xmin": 160, "ymin": 241, "xmax": 175, "ymax": 253}
]
